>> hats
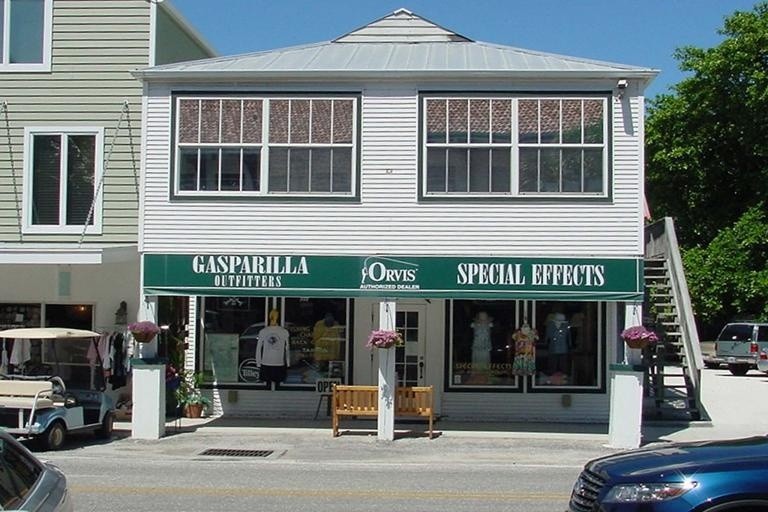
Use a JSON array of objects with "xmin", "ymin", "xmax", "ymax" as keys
[
  {"xmin": 269, "ymin": 309, "xmax": 279, "ymax": 325},
  {"xmin": 550, "ymin": 312, "xmax": 569, "ymax": 323},
  {"xmin": 473, "ymin": 312, "xmax": 493, "ymax": 322}
]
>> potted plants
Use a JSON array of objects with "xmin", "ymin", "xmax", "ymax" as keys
[{"xmin": 173, "ymin": 365, "xmax": 212, "ymax": 419}]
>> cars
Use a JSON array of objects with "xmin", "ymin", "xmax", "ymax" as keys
[
  {"xmin": 0, "ymin": 427, "xmax": 72, "ymax": 511},
  {"xmin": 755, "ymin": 347, "xmax": 767, "ymax": 375},
  {"xmin": 712, "ymin": 323, "xmax": 767, "ymax": 376},
  {"xmin": 491, "ymin": 332, "xmax": 572, "ymax": 376},
  {"xmin": 238, "ymin": 323, "xmax": 303, "ymax": 368},
  {"xmin": 566, "ymin": 435, "xmax": 766, "ymax": 511}
]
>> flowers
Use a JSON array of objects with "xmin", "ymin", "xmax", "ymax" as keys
[
  {"xmin": 619, "ymin": 325, "xmax": 661, "ymax": 344},
  {"xmin": 365, "ymin": 327, "xmax": 405, "ymax": 351},
  {"xmin": 127, "ymin": 320, "xmax": 162, "ymax": 333}
]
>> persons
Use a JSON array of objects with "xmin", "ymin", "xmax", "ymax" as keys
[
  {"xmin": 510, "ymin": 323, "xmax": 539, "ymax": 376},
  {"xmin": 255, "ymin": 310, "xmax": 290, "ymax": 382},
  {"xmin": 470, "ymin": 312, "xmax": 494, "ymax": 366},
  {"xmin": 310, "ymin": 311, "xmax": 341, "ymax": 373},
  {"xmin": 540, "ymin": 312, "xmax": 571, "ymax": 376}
]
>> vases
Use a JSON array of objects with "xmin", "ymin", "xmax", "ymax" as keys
[
  {"xmin": 133, "ymin": 332, "xmax": 157, "ymax": 344},
  {"xmin": 629, "ymin": 338, "xmax": 649, "ymax": 349},
  {"xmin": 373, "ymin": 339, "xmax": 397, "ymax": 351}
]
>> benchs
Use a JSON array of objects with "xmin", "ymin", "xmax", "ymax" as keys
[{"xmin": 331, "ymin": 382, "xmax": 438, "ymax": 440}]
